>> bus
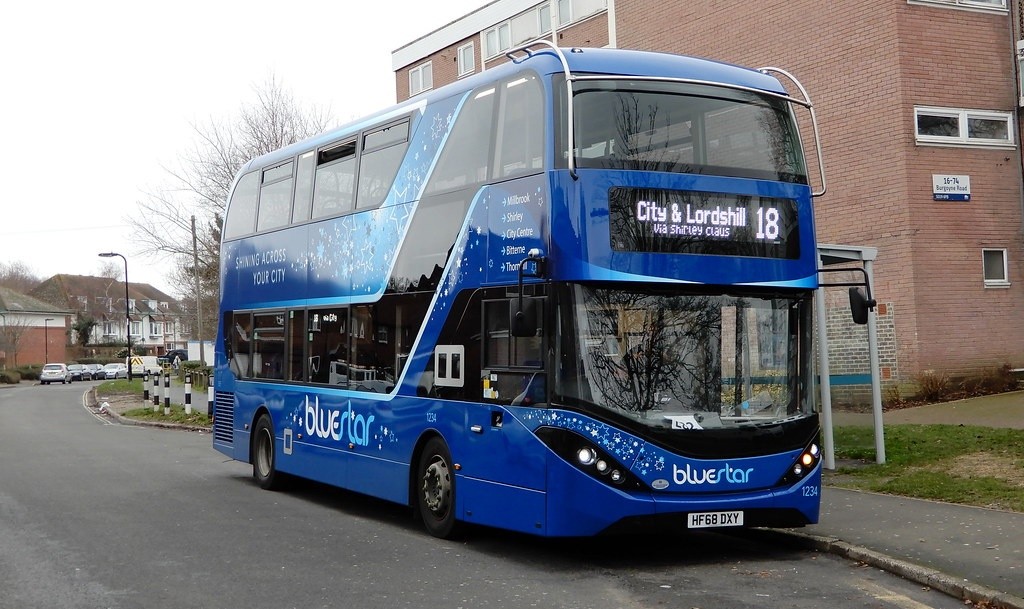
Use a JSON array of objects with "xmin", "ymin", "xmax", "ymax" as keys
[{"xmin": 213, "ymin": 38, "xmax": 881, "ymax": 556}]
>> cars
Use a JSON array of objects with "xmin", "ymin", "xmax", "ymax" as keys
[
  {"xmin": 103, "ymin": 363, "xmax": 129, "ymax": 379},
  {"xmin": 40, "ymin": 363, "xmax": 72, "ymax": 384},
  {"xmin": 67, "ymin": 364, "xmax": 92, "ymax": 381},
  {"xmin": 87, "ymin": 364, "xmax": 106, "ymax": 380}
]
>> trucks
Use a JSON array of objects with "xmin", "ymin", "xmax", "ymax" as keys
[{"xmin": 129, "ymin": 356, "xmax": 163, "ymax": 381}]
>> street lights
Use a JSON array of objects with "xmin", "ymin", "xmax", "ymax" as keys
[
  {"xmin": 98, "ymin": 251, "xmax": 134, "ymax": 382},
  {"xmin": 45, "ymin": 318, "xmax": 56, "ymax": 363}
]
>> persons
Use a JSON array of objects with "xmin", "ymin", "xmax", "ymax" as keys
[
  {"xmin": 617, "ymin": 324, "xmax": 713, "ymax": 391},
  {"xmin": 520, "ymin": 322, "xmax": 545, "ymax": 404}
]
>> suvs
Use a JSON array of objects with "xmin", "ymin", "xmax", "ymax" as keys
[{"xmin": 159, "ymin": 349, "xmax": 188, "ymax": 366}]
